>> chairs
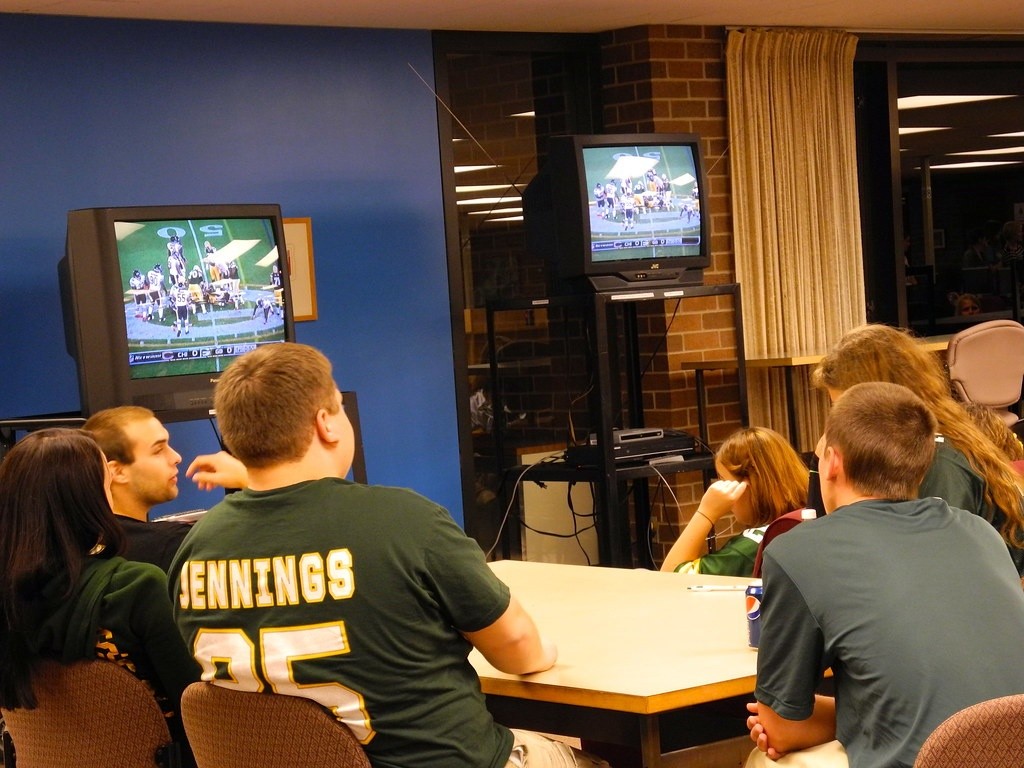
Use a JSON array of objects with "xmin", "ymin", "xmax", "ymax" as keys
[
  {"xmin": 0, "ymin": 656, "xmax": 175, "ymax": 766},
  {"xmin": 751, "ymin": 509, "xmax": 817, "ymax": 580},
  {"xmin": 911, "ymin": 693, "xmax": 1024, "ymax": 766},
  {"xmin": 181, "ymin": 679, "xmax": 371, "ymax": 767}
]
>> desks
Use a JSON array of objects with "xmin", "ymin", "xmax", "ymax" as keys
[
  {"xmin": 469, "ymin": 558, "xmax": 836, "ymax": 766},
  {"xmin": 679, "ymin": 332, "xmax": 955, "ymax": 552}
]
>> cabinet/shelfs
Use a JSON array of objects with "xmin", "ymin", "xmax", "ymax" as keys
[
  {"xmin": 484, "ymin": 283, "xmax": 751, "ymax": 568},
  {"xmin": 0, "ymin": 390, "xmax": 368, "ymax": 482}
]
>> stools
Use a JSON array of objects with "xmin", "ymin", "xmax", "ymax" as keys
[{"xmin": 945, "ymin": 317, "xmax": 1024, "ymax": 426}]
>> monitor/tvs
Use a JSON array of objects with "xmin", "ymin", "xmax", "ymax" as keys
[
  {"xmin": 58, "ymin": 207, "xmax": 297, "ymax": 422},
  {"xmin": 522, "ymin": 132, "xmax": 712, "ymax": 292}
]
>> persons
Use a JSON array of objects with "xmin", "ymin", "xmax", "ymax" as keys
[
  {"xmin": 164, "ymin": 342, "xmax": 616, "ymax": 767},
  {"xmin": 130, "ymin": 226, "xmax": 286, "ymax": 324},
  {"xmin": 82, "ymin": 403, "xmax": 195, "ymax": 575},
  {"xmin": 809, "ymin": 325, "xmax": 1022, "ymax": 577},
  {"xmin": 746, "ymin": 380, "xmax": 1022, "ymax": 768},
  {"xmin": 659, "ymin": 426, "xmax": 810, "ymax": 576},
  {"xmin": 591, "ymin": 152, "xmax": 708, "ymax": 238},
  {"xmin": 0, "ymin": 426, "xmax": 172, "ymax": 768}
]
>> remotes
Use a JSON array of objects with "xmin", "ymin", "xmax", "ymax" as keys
[{"xmin": 645, "ymin": 455, "xmax": 685, "ymax": 465}]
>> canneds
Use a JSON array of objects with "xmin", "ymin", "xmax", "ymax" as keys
[{"xmin": 744, "ymin": 583, "xmax": 763, "ymax": 649}]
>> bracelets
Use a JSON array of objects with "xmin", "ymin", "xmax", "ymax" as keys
[{"xmin": 695, "ymin": 509, "xmax": 715, "ymax": 527}]
